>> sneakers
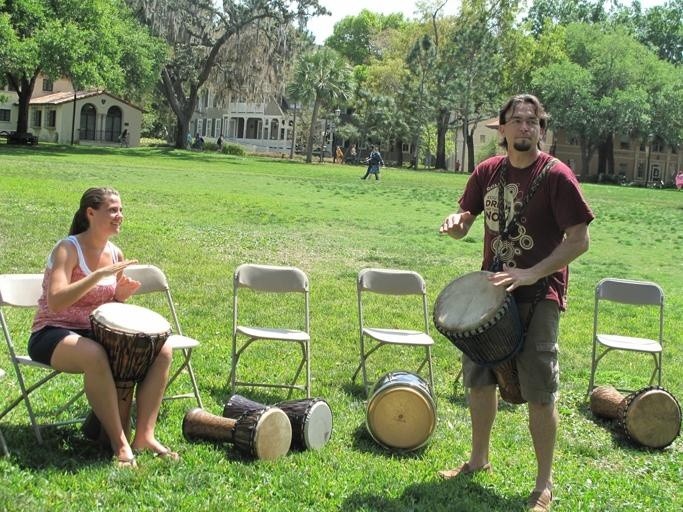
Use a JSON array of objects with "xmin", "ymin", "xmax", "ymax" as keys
[
  {"xmin": 436, "ymin": 464, "xmax": 493, "ymax": 483},
  {"xmin": 526, "ymin": 488, "xmax": 554, "ymax": 512}
]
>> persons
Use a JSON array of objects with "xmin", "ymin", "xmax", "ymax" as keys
[
  {"xmin": 361, "ymin": 146, "xmax": 384, "ymax": 181},
  {"xmin": 120, "ymin": 129, "xmax": 130, "ymax": 148},
  {"xmin": 187, "ymin": 133, "xmax": 205, "ymax": 152},
  {"xmin": 336, "ymin": 146, "xmax": 343, "ymax": 164},
  {"xmin": 345, "ymin": 144, "xmax": 357, "ymax": 165},
  {"xmin": 438, "ymin": 94, "xmax": 596, "ymax": 512},
  {"xmin": 27, "ymin": 187, "xmax": 180, "ymax": 470},
  {"xmin": 217, "ymin": 134, "xmax": 224, "ymax": 150},
  {"xmin": 407, "ymin": 153, "xmax": 461, "ymax": 172}
]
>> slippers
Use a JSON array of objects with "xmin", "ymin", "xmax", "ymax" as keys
[
  {"xmin": 114, "ymin": 448, "xmax": 140, "ymax": 473},
  {"xmin": 133, "ymin": 447, "xmax": 181, "ymax": 467}
]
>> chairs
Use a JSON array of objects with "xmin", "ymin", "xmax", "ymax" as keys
[
  {"xmin": 122, "ymin": 264, "xmax": 204, "ymax": 410},
  {"xmin": 225, "ymin": 263, "xmax": 310, "ymax": 400},
  {"xmin": 0, "ymin": 274, "xmax": 86, "ymax": 445},
  {"xmin": 352, "ymin": 268, "xmax": 435, "ymax": 399},
  {"xmin": 584, "ymin": 278, "xmax": 664, "ymax": 402}
]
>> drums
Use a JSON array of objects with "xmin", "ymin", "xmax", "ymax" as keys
[
  {"xmin": 80, "ymin": 303, "xmax": 172, "ymax": 453},
  {"xmin": 589, "ymin": 383, "xmax": 680, "ymax": 448},
  {"xmin": 223, "ymin": 394, "xmax": 333, "ymax": 451},
  {"xmin": 366, "ymin": 371, "xmax": 437, "ymax": 451},
  {"xmin": 181, "ymin": 407, "xmax": 292, "ymax": 457},
  {"xmin": 432, "ymin": 271, "xmax": 523, "ymax": 404}
]
